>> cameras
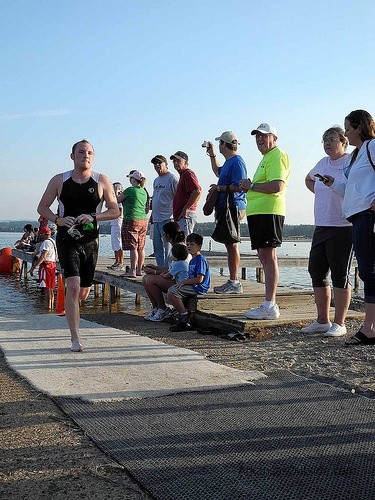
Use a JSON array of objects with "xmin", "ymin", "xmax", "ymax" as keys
[{"xmin": 202, "ymin": 140, "xmax": 209, "ymax": 148}]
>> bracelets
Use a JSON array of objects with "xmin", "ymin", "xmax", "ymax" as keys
[
  {"xmin": 55, "ymin": 216, "xmax": 62, "ymax": 225},
  {"xmin": 250, "ymin": 183, "xmax": 253, "ymax": 189},
  {"xmin": 217, "ymin": 185, "xmax": 220, "ymax": 191}
]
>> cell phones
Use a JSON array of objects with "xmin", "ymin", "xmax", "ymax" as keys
[{"xmin": 313, "ymin": 173, "xmax": 328, "ymax": 182}]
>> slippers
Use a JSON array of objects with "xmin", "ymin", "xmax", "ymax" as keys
[
  {"xmin": 345, "ymin": 331, "xmax": 375, "ymax": 345},
  {"xmin": 122, "ymin": 272, "xmax": 142, "ymax": 278}
]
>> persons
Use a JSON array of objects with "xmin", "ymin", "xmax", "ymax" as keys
[
  {"xmin": 167, "ymin": 233, "xmax": 210, "ymax": 331},
  {"xmin": 160, "ymin": 243, "xmax": 189, "ymax": 315},
  {"xmin": 14, "ymin": 223, "xmax": 57, "ymax": 264},
  {"xmin": 115, "ymin": 170, "xmax": 147, "ymax": 278},
  {"xmin": 38, "ymin": 215, "xmax": 48, "ymax": 227},
  {"xmin": 143, "ymin": 222, "xmax": 192, "ymax": 322},
  {"xmin": 37, "ymin": 140, "xmax": 119, "ymax": 352},
  {"xmin": 107, "ymin": 182, "xmax": 123, "ymax": 270},
  {"xmin": 319, "ymin": 109, "xmax": 375, "ymax": 345},
  {"xmin": 170, "ymin": 150, "xmax": 201, "ymax": 239},
  {"xmin": 27, "ymin": 226, "xmax": 58, "ymax": 308},
  {"xmin": 239, "ymin": 122, "xmax": 290, "ymax": 319},
  {"xmin": 305, "ymin": 127, "xmax": 355, "ymax": 336},
  {"xmin": 206, "ymin": 131, "xmax": 247, "ymax": 292},
  {"xmin": 150, "ymin": 155, "xmax": 177, "ymax": 268}
]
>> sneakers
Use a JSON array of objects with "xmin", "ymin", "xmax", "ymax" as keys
[
  {"xmin": 149, "ymin": 307, "xmax": 173, "ymax": 321},
  {"xmin": 144, "ymin": 307, "xmax": 158, "ymax": 320},
  {"xmin": 324, "ymin": 322, "xmax": 347, "ymax": 336},
  {"xmin": 214, "ymin": 280, "xmax": 243, "ymax": 294},
  {"xmin": 246, "ymin": 304, "xmax": 280, "ymax": 319},
  {"xmin": 300, "ymin": 320, "xmax": 332, "ymax": 332}
]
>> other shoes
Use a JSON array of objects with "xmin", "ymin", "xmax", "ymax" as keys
[
  {"xmin": 106, "ymin": 262, "xmax": 124, "ymax": 270},
  {"xmin": 172, "ymin": 312, "xmax": 181, "ymax": 323},
  {"xmin": 180, "ymin": 310, "xmax": 189, "ymax": 322}
]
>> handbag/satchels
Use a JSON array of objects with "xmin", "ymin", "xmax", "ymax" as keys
[{"xmin": 211, "ymin": 186, "xmax": 241, "ymax": 244}]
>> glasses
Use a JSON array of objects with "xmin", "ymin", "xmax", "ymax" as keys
[
  {"xmin": 321, "ymin": 137, "xmax": 341, "ymax": 143},
  {"xmin": 256, "ymin": 134, "xmax": 273, "ymax": 138}
]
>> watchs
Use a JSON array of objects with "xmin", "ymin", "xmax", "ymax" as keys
[{"xmin": 90, "ymin": 213, "xmax": 96, "ymax": 221}]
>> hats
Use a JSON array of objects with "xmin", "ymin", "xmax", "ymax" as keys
[
  {"xmin": 170, "ymin": 151, "xmax": 188, "ymax": 160},
  {"xmin": 126, "ymin": 170, "xmax": 144, "ymax": 182},
  {"xmin": 251, "ymin": 123, "xmax": 278, "ymax": 138},
  {"xmin": 215, "ymin": 131, "xmax": 238, "ymax": 145},
  {"xmin": 151, "ymin": 155, "xmax": 166, "ymax": 163},
  {"xmin": 38, "ymin": 226, "xmax": 50, "ymax": 234}
]
